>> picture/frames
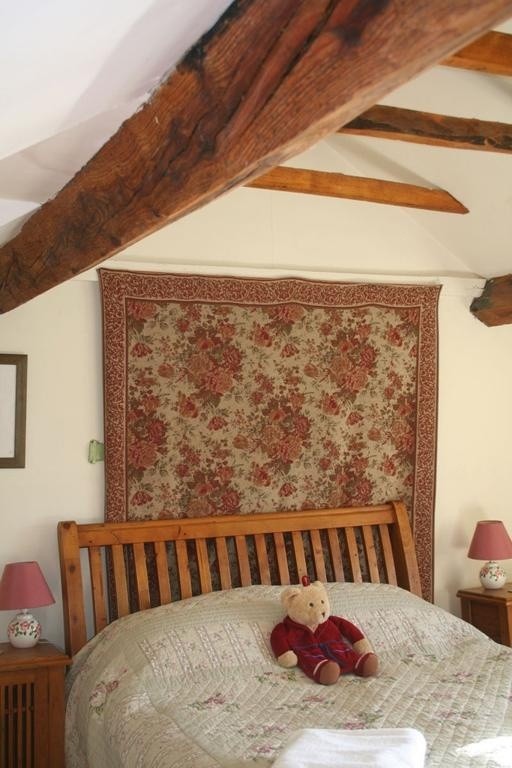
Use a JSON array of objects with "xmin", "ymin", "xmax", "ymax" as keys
[{"xmin": 0, "ymin": 353, "xmax": 28, "ymax": 470}]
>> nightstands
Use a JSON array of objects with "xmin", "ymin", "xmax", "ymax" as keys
[
  {"xmin": 0, "ymin": 640, "xmax": 73, "ymax": 768},
  {"xmin": 456, "ymin": 584, "xmax": 511, "ymax": 647}
]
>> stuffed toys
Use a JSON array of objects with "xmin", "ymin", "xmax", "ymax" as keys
[{"xmin": 270, "ymin": 576, "xmax": 378, "ymax": 685}]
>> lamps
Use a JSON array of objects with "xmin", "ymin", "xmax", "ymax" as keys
[
  {"xmin": 1, "ymin": 560, "xmax": 57, "ymax": 650},
  {"xmin": 466, "ymin": 520, "xmax": 510, "ymax": 590}
]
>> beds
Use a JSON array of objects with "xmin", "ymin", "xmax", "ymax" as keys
[{"xmin": 57, "ymin": 501, "xmax": 511, "ymax": 768}]
